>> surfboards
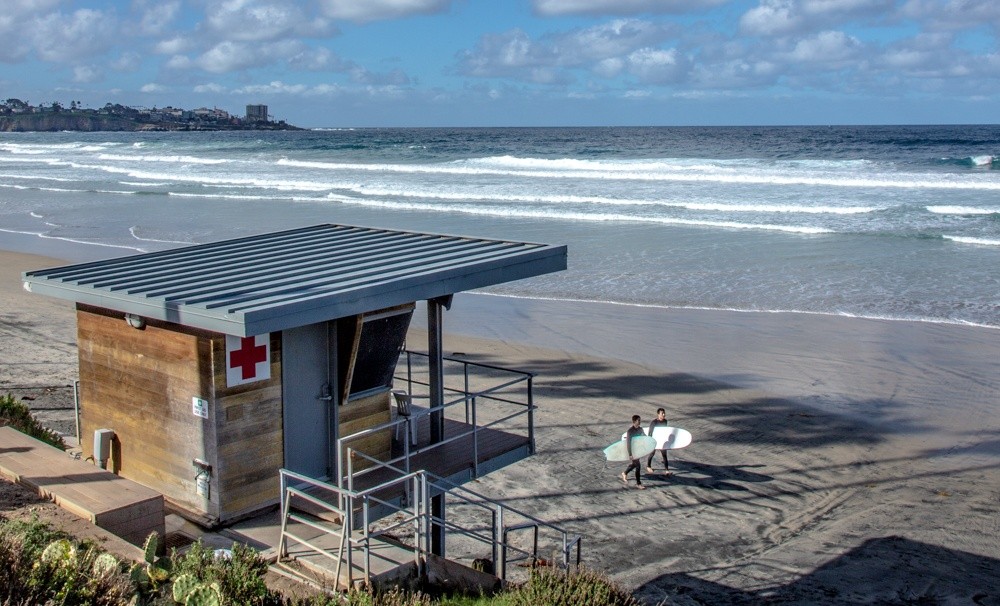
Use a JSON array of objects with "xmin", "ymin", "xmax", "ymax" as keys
[
  {"xmin": 621, "ymin": 426, "xmax": 692, "ymax": 450},
  {"xmin": 603, "ymin": 436, "xmax": 657, "ymax": 462}
]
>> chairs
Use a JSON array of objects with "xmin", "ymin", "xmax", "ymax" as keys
[{"xmin": 393, "ymin": 390, "xmax": 429, "ymax": 445}]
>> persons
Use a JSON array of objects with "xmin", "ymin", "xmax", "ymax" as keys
[
  {"xmin": 622, "ymin": 415, "xmax": 645, "ymax": 490},
  {"xmin": 647, "ymin": 408, "xmax": 673, "ymax": 473}
]
